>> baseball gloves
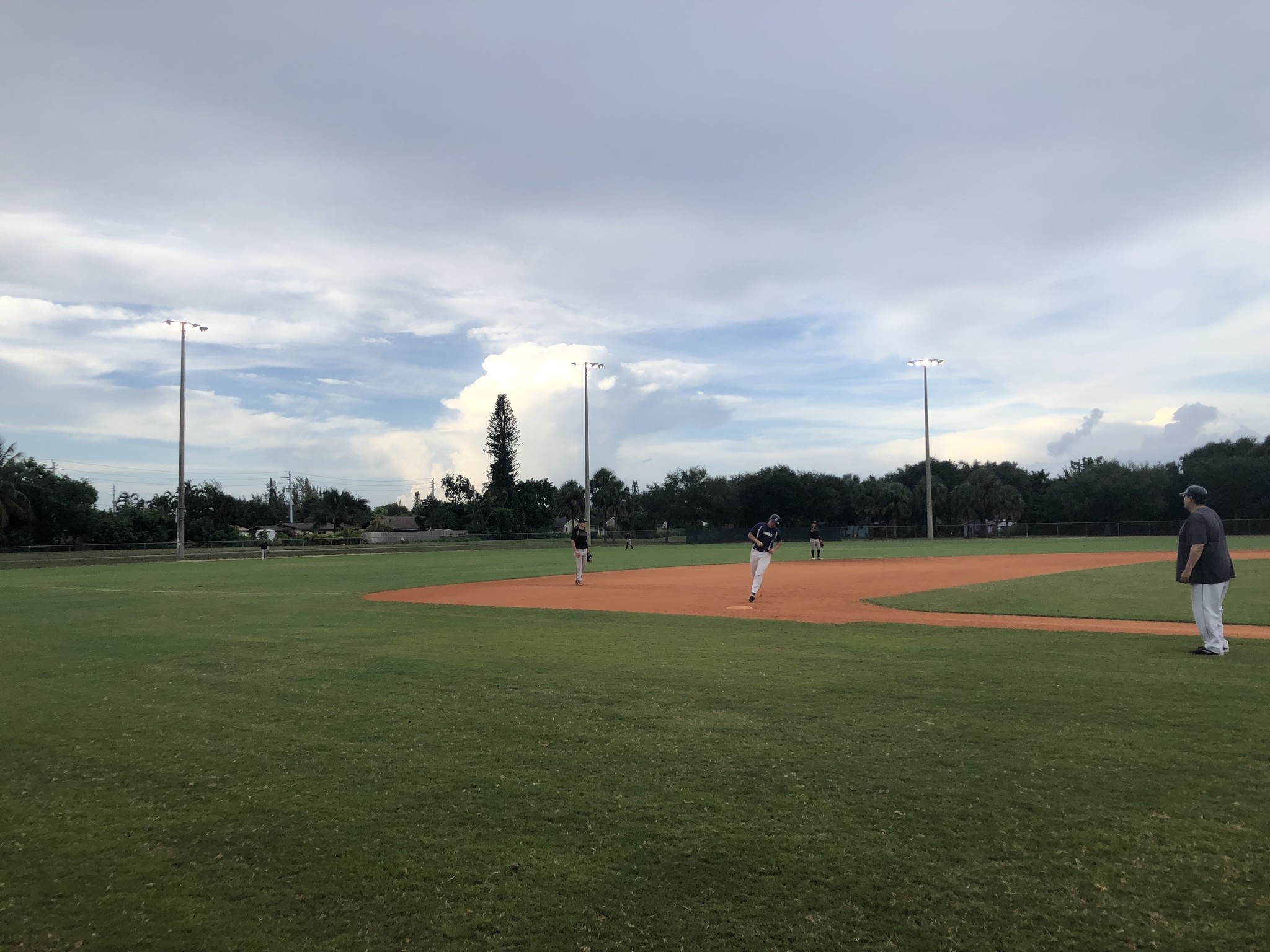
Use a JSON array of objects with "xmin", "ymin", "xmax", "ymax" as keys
[
  {"xmin": 820, "ymin": 542, "xmax": 824, "ymax": 548},
  {"xmin": 626, "ymin": 541, "xmax": 629, "ymax": 545},
  {"xmin": 586, "ymin": 552, "xmax": 592, "ymax": 564}
]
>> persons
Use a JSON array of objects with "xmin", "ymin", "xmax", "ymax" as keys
[
  {"xmin": 259, "ymin": 539, "xmax": 269, "ymax": 560},
  {"xmin": 748, "ymin": 514, "xmax": 782, "ymax": 602},
  {"xmin": 624, "ymin": 531, "xmax": 634, "ymax": 550},
  {"xmin": 1175, "ymin": 484, "xmax": 1235, "ymax": 655},
  {"xmin": 809, "ymin": 521, "xmax": 824, "ymax": 560},
  {"xmin": 570, "ymin": 518, "xmax": 592, "ymax": 586}
]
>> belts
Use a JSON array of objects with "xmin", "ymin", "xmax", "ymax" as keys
[
  {"xmin": 752, "ymin": 547, "xmax": 769, "ymax": 552},
  {"xmin": 579, "ymin": 548, "xmax": 586, "ymax": 549}
]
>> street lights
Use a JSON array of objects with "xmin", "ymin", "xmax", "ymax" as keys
[
  {"xmin": 159, "ymin": 318, "xmax": 209, "ymax": 560},
  {"xmin": 569, "ymin": 361, "xmax": 605, "ymax": 544},
  {"xmin": 906, "ymin": 357, "xmax": 944, "ymax": 539}
]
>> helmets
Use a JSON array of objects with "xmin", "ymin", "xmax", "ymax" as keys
[{"xmin": 1179, "ymin": 486, "xmax": 1207, "ymax": 503}]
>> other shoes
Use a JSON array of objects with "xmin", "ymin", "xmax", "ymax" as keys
[
  {"xmin": 817, "ymin": 557, "xmax": 824, "ymax": 560},
  {"xmin": 624, "ymin": 549, "xmax": 627, "ymax": 550},
  {"xmin": 812, "ymin": 558, "xmax": 816, "ymax": 560},
  {"xmin": 632, "ymin": 548, "xmax": 634, "ymax": 550}
]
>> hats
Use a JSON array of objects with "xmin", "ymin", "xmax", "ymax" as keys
[
  {"xmin": 812, "ymin": 521, "xmax": 817, "ymax": 524},
  {"xmin": 578, "ymin": 519, "xmax": 588, "ymax": 523},
  {"xmin": 769, "ymin": 514, "xmax": 780, "ymax": 524}
]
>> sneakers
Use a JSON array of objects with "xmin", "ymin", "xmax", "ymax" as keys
[
  {"xmin": 577, "ymin": 581, "xmax": 583, "ymax": 586},
  {"xmin": 1189, "ymin": 649, "xmax": 1224, "ymax": 656},
  {"xmin": 575, "ymin": 580, "xmax": 584, "ymax": 585},
  {"xmin": 749, "ymin": 595, "xmax": 755, "ymax": 602},
  {"xmin": 1198, "ymin": 646, "xmax": 1229, "ymax": 653}
]
